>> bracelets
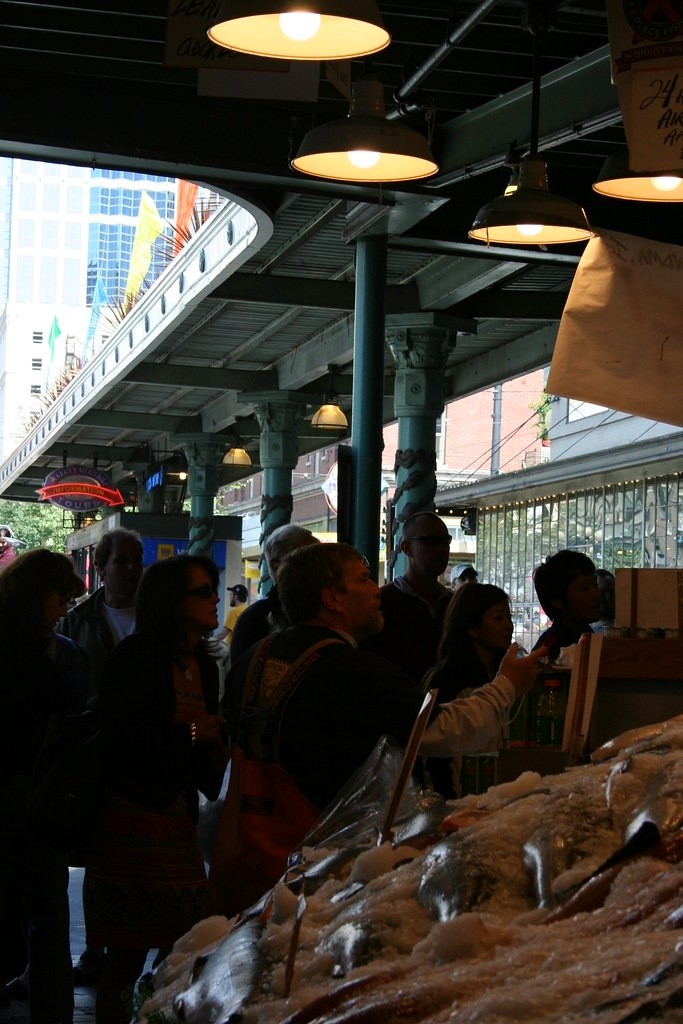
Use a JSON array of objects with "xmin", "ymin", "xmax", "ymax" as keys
[{"xmin": 495, "ymin": 672, "xmax": 513, "ymax": 690}]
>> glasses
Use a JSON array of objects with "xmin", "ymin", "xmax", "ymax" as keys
[
  {"xmin": 405, "ymin": 534, "xmax": 452, "ymax": 547},
  {"xmin": 185, "ymin": 583, "xmax": 218, "ymax": 602}
]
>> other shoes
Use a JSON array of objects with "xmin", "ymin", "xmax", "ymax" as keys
[
  {"xmin": 74, "ymin": 962, "xmax": 99, "ymax": 987},
  {"xmin": 5, "ymin": 977, "xmax": 28, "ymax": 1001}
]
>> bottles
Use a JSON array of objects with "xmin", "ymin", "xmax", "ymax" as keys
[{"xmin": 537, "ymin": 680, "xmax": 564, "ymax": 749}]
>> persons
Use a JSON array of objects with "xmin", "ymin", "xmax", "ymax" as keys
[
  {"xmin": 447, "ymin": 562, "xmax": 479, "ymax": 593},
  {"xmin": 362, "ymin": 510, "xmax": 461, "ymax": 789},
  {"xmin": 421, "ymin": 583, "xmax": 514, "ymax": 800},
  {"xmin": 588, "ymin": 569, "xmax": 617, "ymax": 632},
  {"xmin": 529, "ymin": 550, "xmax": 603, "ymax": 719},
  {"xmin": 0, "ymin": 524, "xmax": 320, "ymax": 1024},
  {"xmin": 235, "ymin": 543, "xmax": 548, "ymax": 824}
]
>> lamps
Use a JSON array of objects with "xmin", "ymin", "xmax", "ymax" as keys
[
  {"xmin": 290, "ymin": 16, "xmax": 440, "ymax": 183},
  {"xmin": 221, "ymin": 416, "xmax": 253, "ymax": 467},
  {"xmin": 311, "ymin": 375, "xmax": 347, "ymax": 432},
  {"xmin": 592, "ymin": 142, "xmax": 683, "ymax": 203},
  {"xmin": 504, "ymin": 168, "xmax": 519, "ymax": 196},
  {"xmin": 471, "ymin": 34, "xmax": 589, "ymax": 246},
  {"xmin": 208, "ymin": 0, "xmax": 388, "ymax": 63}
]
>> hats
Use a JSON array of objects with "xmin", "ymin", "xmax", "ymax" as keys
[{"xmin": 227, "ymin": 585, "xmax": 249, "ymax": 598}]
